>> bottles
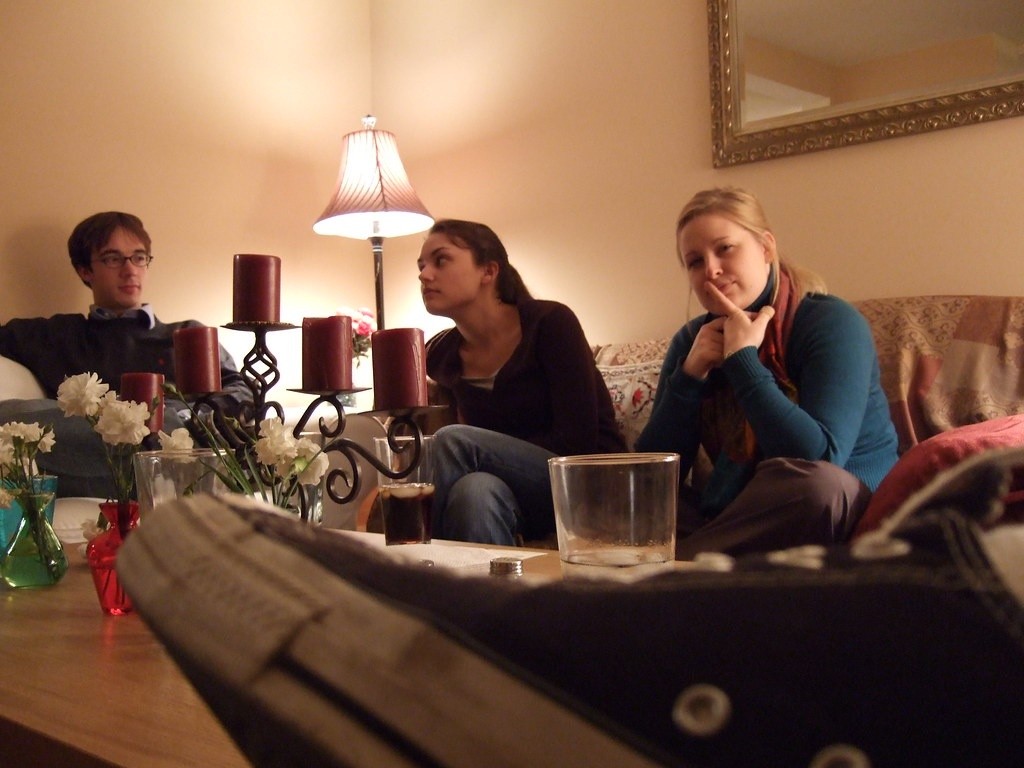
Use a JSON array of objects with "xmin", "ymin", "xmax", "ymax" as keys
[{"xmin": 488, "ymin": 557, "xmax": 521, "ymax": 579}]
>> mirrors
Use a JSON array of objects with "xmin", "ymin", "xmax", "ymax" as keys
[{"xmin": 707, "ymin": 0, "xmax": 1024, "ymax": 169}]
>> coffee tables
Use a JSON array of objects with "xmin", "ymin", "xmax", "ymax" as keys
[{"xmin": 0, "ymin": 525, "xmax": 693, "ymax": 768}]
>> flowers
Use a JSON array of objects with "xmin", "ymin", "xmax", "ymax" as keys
[
  {"xmin": 161, "ymin": 383, "xmax": 330, "ymax": 509},
  {"xmin": 56, "ymin": 371, "xmax": 200, "ymax": 501},
  {"xmin": 0, "ymin": 422, "xmax": 55, "ymax": 509},
  {"xmin": 338, "ymin": 306, "xmax": 377, "ymax": 370}
]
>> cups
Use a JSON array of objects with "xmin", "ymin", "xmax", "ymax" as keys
[
  {"xmin": 374, "ymin": 434, "xmax": 438, "ymax": 545},
  {"xmin": 548, "ymin": 452, "xmax": 679, "ymax": 578},
  {"xmin": 133, "ymin": 449, "xmax": 238, "ymax": 529},
  {"xmin": 0, "ymin": 474, "xmax": 57, "ymax": 551}
]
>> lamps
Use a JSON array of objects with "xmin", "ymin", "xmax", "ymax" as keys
[{"xmin": 313, "ymin": 113, "xmax": 439, "ymax": 330}]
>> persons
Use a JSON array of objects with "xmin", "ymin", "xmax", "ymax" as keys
[
  {"xmin": 418, "ymin": 219, "xmax": 629, "ymax": 547},
  {"xmin": 634, "ymin": 186, "xmax": 899, "ymax": 561},
  {"xmin": 0, "ymin": 211, "xmax": 258, "ymax": 500}
]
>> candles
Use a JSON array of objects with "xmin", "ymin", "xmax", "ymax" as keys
[
  {"xmin": 232, "ymin": 253, "xmax": 281, "ymax": 322},
  {"xmin": 119, "ymin": 372, "xmax": 166, "ymax": 432},
  {"xmin": 172, "ymin": 326, "xmax": 222, "ymax": 395},
  {"xmin": 302, "ymin": 315, "xmax": 353, "ymax": 391},
  {"xmin": 371, "ymin": 327, "xmax": 428, "ymax": 410}
]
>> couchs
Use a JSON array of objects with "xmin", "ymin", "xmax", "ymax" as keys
[{"xmin": 319, "ymin": 295, "xmax": 1024, "ymax": 530}]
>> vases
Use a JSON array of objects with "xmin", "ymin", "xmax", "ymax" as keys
[
  {"xmin": 0, "ymin": 492, "xmax": 69, "ymax": 592},
  {"xmin": 86, "ymin": 503, "xmax": 142, "ymax": 615},
  {"xmin": 0, "ymin": 474, "xmax": 58, "ymax": 562}
]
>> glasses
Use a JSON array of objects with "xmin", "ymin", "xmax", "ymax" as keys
[{"xmin": 83, "ymin": 251, "xmax": 153, "ymax": 268}]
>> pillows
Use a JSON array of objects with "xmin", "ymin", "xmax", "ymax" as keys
[
  {"xmin": 594, "ymin": 360, "xmax": 665, "ymax": 452},
  {"xmin": 853, "ymin": 415, "xmax": 1024, "ymax": 540}
]
